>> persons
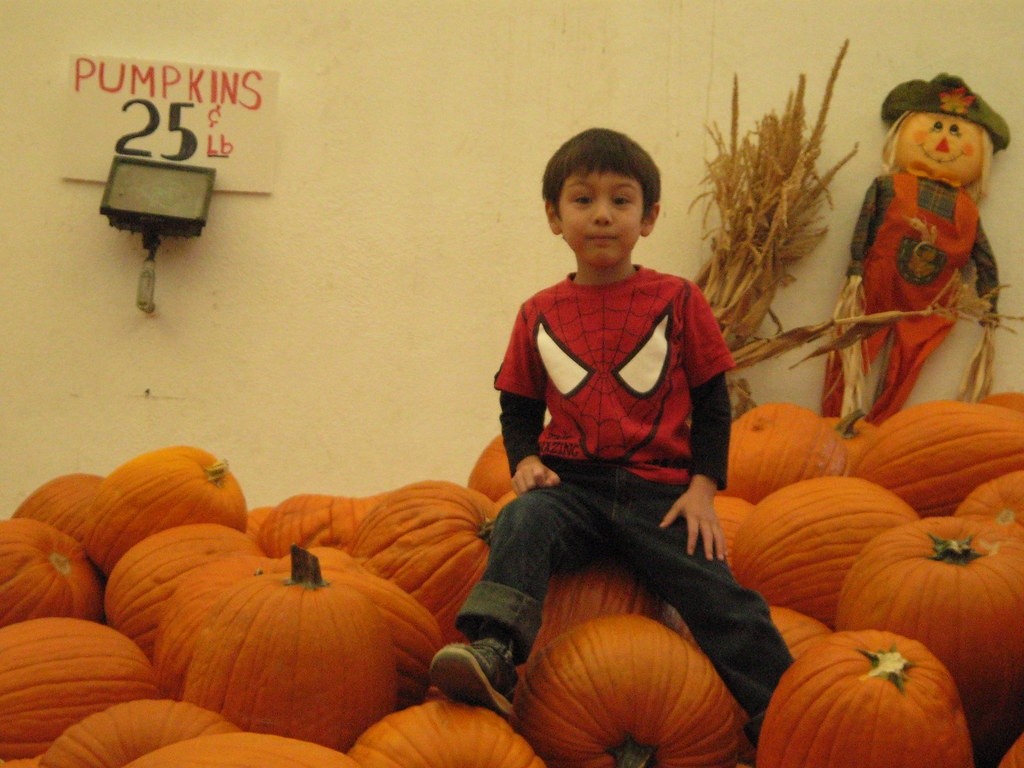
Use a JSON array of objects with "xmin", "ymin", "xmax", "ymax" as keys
[
  {"xmin": 822, "ymin": 71, "xmax": 1011, "ymax": 420},
  {"xmin": 430, "ymin": 129, "xmax": 795, "ymax": 750}
]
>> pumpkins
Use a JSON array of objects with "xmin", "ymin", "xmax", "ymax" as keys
[{"xmin": 0, "ymin": 393, "xmax": 1024, "ymax": 768}]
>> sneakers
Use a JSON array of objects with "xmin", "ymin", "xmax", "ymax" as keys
[{"xmin": 429, "ymin": 638, "xmax": 518, "ymax": 716}]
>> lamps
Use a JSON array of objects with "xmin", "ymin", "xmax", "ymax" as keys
[{"xmin": 100, "ymin": 156, "xmax": 216, "ymax": 314}]
